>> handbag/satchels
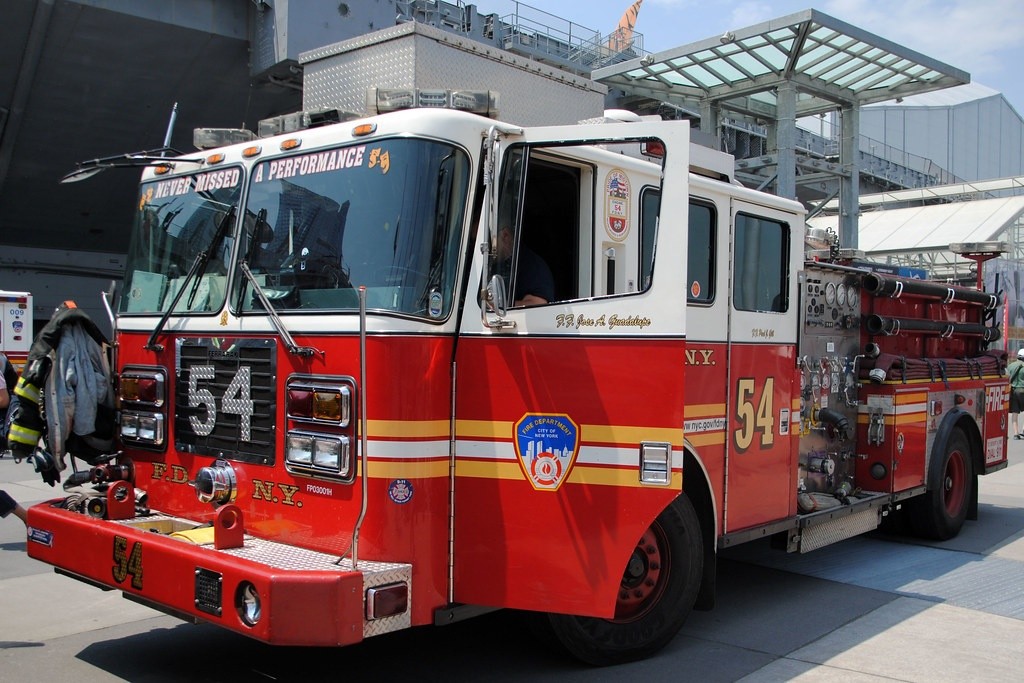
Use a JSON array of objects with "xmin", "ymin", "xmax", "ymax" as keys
[
  {"xmin": 65, "ymin": 401, "xmax": 118, "ymax": 465},
  {"xmin": 0, "ymin": 354, "xmax": 18, "ymax": 391}
]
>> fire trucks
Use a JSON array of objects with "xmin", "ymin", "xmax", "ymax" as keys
[{"xmin": 22, "ymin": 87, "xmax": 1012, "ymax": 670}]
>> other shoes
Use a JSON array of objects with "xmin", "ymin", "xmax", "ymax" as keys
[
  {"xmin": 1019, "ymin": 434, "xmax": 1024, "ymax": 439},
  {"xmin": 1014, "ymin": 434, "xmax": 1020, "ymax": 440}
]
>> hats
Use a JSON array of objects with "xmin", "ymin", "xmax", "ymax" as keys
[{"xmin": 1017, "ymin": 348, "xmax": 1024, "ymax": 359}]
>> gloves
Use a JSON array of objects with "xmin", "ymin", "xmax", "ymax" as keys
[{"xmin": 27, "ymin": 447, "xmax": 61, "ymax": 486}]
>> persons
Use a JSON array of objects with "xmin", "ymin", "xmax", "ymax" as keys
[
  {"xmin": 0, "ymin": 370, "xmax": 27, "ymax": 528},
  {"xmin": 488, "ymin": 216, "xmax": 552, "ymax": 306},
  {"xmin": 1007, "ymin": 348, "xmax": 1024, "ymax": 439}
]
